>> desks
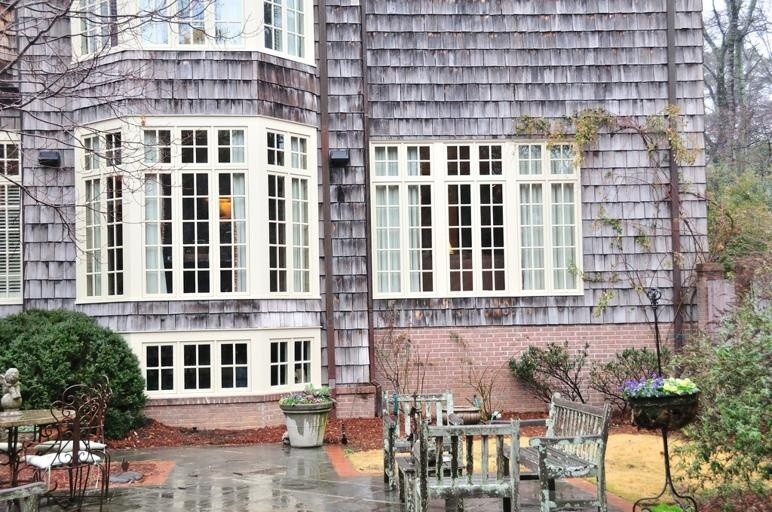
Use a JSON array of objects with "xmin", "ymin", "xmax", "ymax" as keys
[{"xmin": 393, "ymin": 453, "xmax": 467, "ymax": 512}]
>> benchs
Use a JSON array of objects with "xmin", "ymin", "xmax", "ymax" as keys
[{"xmin": 490, "ymin": 392, "xmax": 611, "ymax": 512}]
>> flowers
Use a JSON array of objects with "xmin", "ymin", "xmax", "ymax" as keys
[
  {"xmin": 623, "ymin": 377, "xmax": 699, "ymax": 397},
  {"xmin": 279, "ymin": 384, "xmax": 333, "ymax": 405}
]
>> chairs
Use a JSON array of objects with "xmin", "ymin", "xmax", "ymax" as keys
[
  {"xmin": 382, "ymin": 394, "xmax": 454, "ymax": 492},
  {"xmin": 412, "ymin": 419, "xmax": 522, "ymax": 512},
  {"xmin": 0, "ymin": 373, "xmax": 114, "ymax": 511}
]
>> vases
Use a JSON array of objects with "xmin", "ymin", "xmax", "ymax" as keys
[{"xmin": 281, "ymin": 405, "xmax": 331, "ymax": 448}]
